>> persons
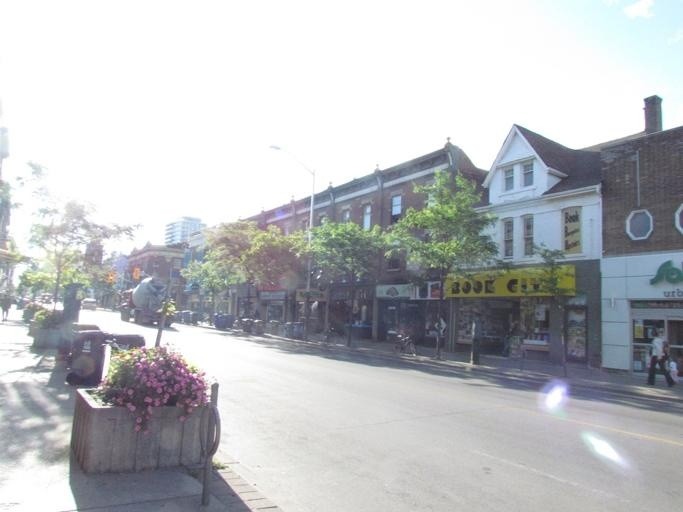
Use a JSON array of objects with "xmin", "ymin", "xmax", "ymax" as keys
[
  {"xmin": 644, "ymin": 325, "xmax": 677, "ymax": 388},
  {"xmin": 0, "ymin": 294, "xmax": 10, "ymax": 322}
]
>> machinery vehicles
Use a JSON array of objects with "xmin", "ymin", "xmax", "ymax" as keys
[{"xmin": 116, "ymin": 277, "xmax": 173, "ymax": 326}]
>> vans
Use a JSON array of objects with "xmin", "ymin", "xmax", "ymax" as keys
[{"xmin": 82, "ymin": 299, "xmax": 97, "ymax": 309}]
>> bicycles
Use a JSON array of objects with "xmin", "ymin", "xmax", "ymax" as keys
[{"xmin": 393, "ymin": 336, "xmax": 416, "ymax": 355}]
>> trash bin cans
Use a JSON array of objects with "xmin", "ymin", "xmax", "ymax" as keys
[
  {"xmin": 253, "ymin": 319, "xmax": 264, "ymax": 335},
  {"xmin": 241, "ymin": 318, "xmax": 252, "ymax": 332},
  {"xmin": 69, "ymin": 330, "xmax": 103, "ymax": 387},
  {"xmin": 343, "ymin": 324, "xmax": 361, "ymax": 347}
]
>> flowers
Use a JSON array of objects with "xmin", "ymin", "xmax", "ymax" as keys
[{"xmin": 98, "ymin": 342, "xmax": 210, "ymax": 438}]
